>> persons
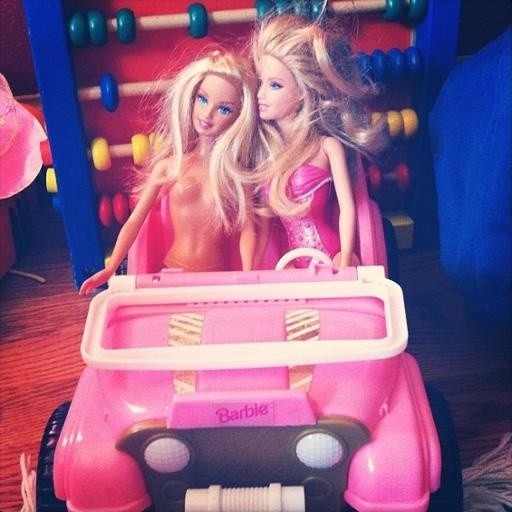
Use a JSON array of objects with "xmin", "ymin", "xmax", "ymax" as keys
[
  {"xmin": 78, "ymin": 42, "xmax": 256, "ymax": 298},
  {"xmin": 237, "ymin": 12, "xmax": 388, "ymax": 270}
]
